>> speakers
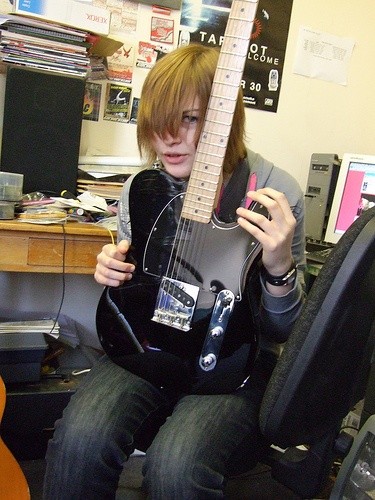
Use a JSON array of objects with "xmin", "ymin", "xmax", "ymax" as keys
[{"xmin": 0, "ymin": 63, "xmax": 85, "ymax": 199}]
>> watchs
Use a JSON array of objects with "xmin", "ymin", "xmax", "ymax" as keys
[{"xmin": 260, "ymin": 260, "xmax": 297, "ymax": 286}]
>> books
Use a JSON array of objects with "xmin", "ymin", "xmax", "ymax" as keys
[
  {"xmin": 77, "ymin": 180, "xmax": 124, "ymax": 200},
  {"xmin": 0, "ymin": 12, "xmax": 124, "ymax": 76},
  {"xmin": 0, "ymin": 320, "xmax": 60, "ymax": 339}
]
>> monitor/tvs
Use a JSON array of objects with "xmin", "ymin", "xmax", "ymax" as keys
[{"xmin": 324, "ymin": 152, "xmax": 375, "ymax": 244}]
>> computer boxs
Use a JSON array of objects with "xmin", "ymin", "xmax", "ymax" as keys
[{"xmin": 304, "ymin": 153, "xmax": 340, "ymax": 246}]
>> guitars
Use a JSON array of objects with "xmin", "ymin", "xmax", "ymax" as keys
[
  {"xmin": 0, "ymin": 374, "xmax": 31, "ymax": 500},
  {"xmin": 95, "ymin": 1, "xmax": 272, "ymax": 395}
]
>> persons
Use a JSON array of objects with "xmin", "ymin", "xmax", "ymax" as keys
[{"xmin": 43, "ymin": 44, "xmax": 306, "ymax": 500}]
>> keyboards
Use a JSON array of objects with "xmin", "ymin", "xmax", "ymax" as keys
[{"xmin": 305, "ymin": 247, "xmax": 334, "ymax": 263}]
